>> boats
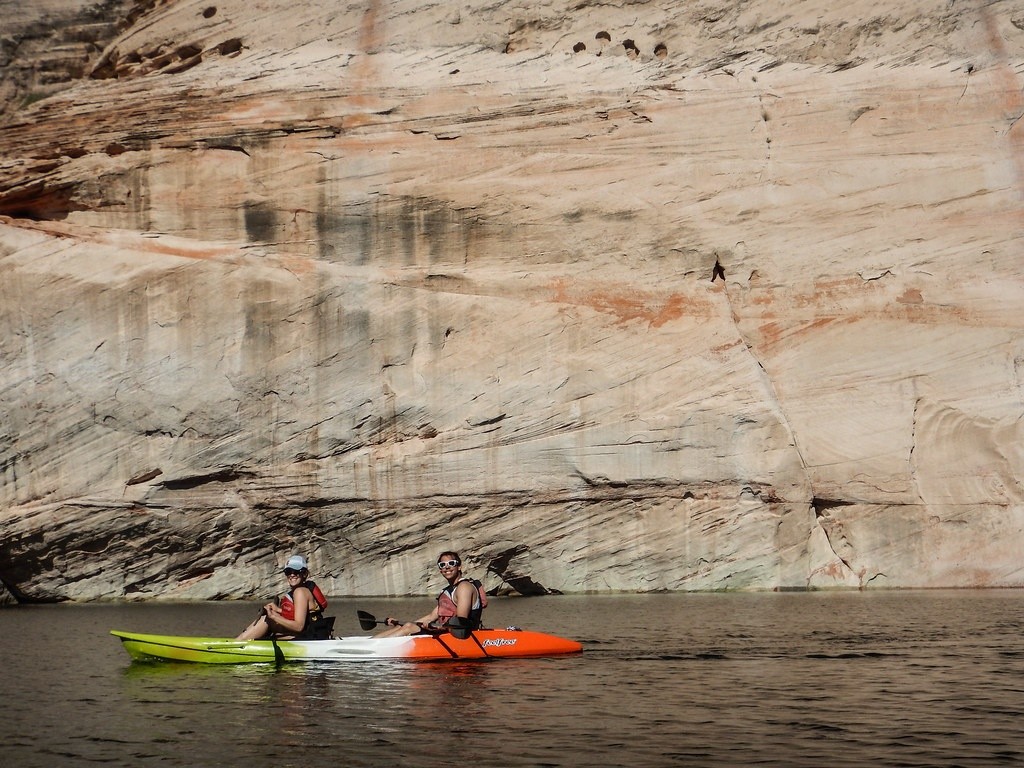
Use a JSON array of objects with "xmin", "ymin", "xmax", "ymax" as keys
[{"xmin": 110, "ymin": 628, "xmax": 583, "ymax": 663}]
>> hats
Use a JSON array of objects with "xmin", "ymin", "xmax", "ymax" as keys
[{"xmin": 282, "ymin": 556, "xmax": 309, "ymax": 569}]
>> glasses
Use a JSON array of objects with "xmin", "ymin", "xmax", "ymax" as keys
[
  {"xmin": 438, "ymin": 560, "xmax": 459, "ymax": 568},
  {"xmin": 285, "ymin": 570, "xmax": 304, "ymax": 576}
]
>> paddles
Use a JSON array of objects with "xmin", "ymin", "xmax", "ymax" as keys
[
  {"xmin": 356, "ymin": 609, "xmax": 475, "ymax": 640},
  {"xmin": 252, "ymin": 593, "xmax": 279, "ymax": 628}
]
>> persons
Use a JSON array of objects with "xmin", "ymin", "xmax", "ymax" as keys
[
  {"xmin": 368, "ymin": 551, "xmax": 482, "ymax": 638},
  {"xmin": 234, "ymin": 555, "xmax": 323, "ymax": 640}
]
